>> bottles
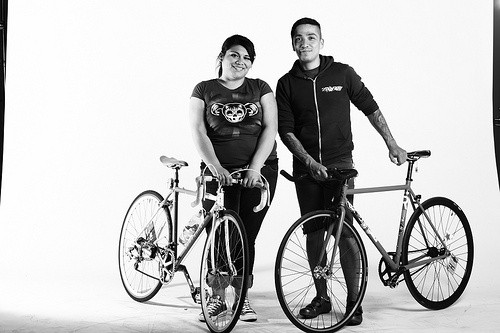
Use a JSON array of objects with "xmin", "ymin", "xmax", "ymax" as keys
[{"xmin": 179, "ymin": 209, "xmax": 205, "ymax": 246}]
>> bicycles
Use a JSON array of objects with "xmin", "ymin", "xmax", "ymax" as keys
[
  {"xmin": 118, "ymin": 156, "xmax": 270, "ymax": 333},
  {"xmin": 275, "ymin": 150, "xmax": 474, "ymax": 333}
]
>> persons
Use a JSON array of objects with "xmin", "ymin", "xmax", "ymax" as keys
[
  {"xmin": 275, "ymin": 18, "xmax": 408, "ymax": 326},
  {"xmin": 191, "ymin": 35, "xmax": 279, "ymax": 322}
]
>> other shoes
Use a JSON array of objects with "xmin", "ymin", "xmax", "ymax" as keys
[
  {"xmin": 344, "ymin": 296, "xmax": 363, "ymax": 325},
  {"xmin": 299, "ymin": 296, "xmax": 332, "ymax": 316}
]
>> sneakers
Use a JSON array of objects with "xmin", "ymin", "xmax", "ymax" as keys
[
  {"xmin": 198, "ymin": 294, "xmax": 228, "ymax": 322},
  {"xmin": 231, "ymin": 297, "xmax": 257, "ymax": 320}
]
